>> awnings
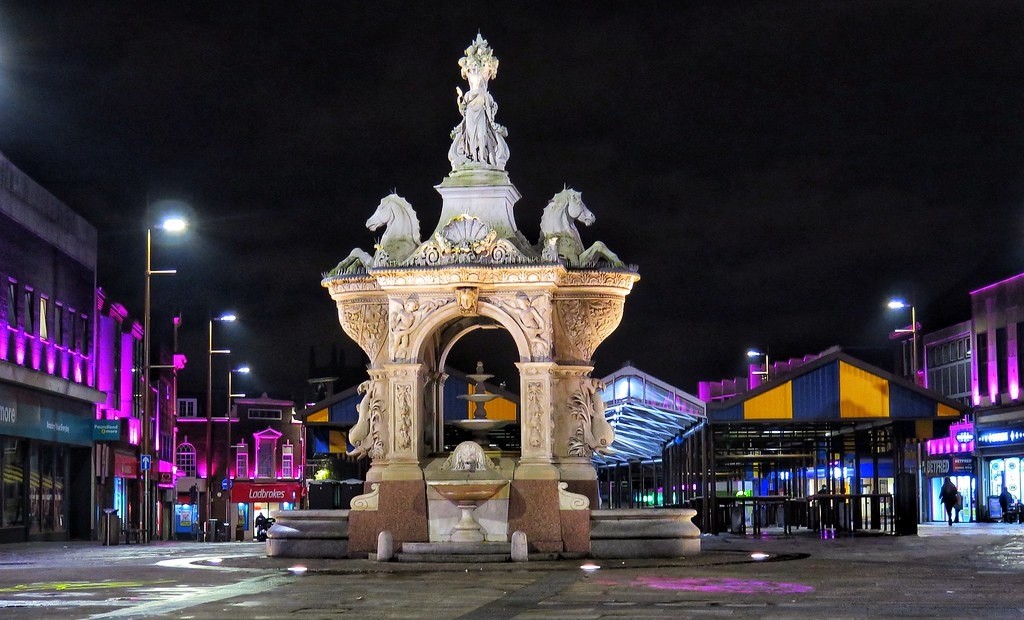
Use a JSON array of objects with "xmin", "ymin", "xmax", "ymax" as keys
[{"xmin": 231, "ymin": 481, "xmax": 300, "ymax": 502}]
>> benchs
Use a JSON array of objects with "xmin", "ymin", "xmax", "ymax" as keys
[{"xmin": 126, "ymin": 529, "xmax": 148, "ymax": 544}]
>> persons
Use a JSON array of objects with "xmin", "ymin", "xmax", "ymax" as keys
[
  {"xmin": 999, "ymin": 486, "xmax": 1014, "ymax": 522},
  {"xmin": 458, "ymin": 288, "xmax": 476, "ymax": 309},
  {"xmin": 461, "ymin": 72, "xmax": 494, "ymax": 162},
  {"xmin": 391, "ymin": 299, "xmax": 416, "ymax": 360},
  {"xmin": 939, "ymin": 477, "xmax": 963, "ymax": 526},
  {"xmin": 498, "ymin": 294, "xmax": 548, "ymax": 354},
  {"xmin": 819, "ymin": 485, "xmax": 827, "ymax": 505}
]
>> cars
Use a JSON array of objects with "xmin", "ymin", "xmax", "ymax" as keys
[{"xmin": 258, "ymin": 517, "xmax": 279, "ymax": 543}]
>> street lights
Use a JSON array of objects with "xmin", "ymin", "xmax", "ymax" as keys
[
  {"xmin": 205, "ymin": 315, "xmax": 236, "ymax": 542},
  {"xmin": 136, "ymin": 218, "xmax": 186, "ymax": 543},
  {"xmin": 889, "ymin": 300, "xmax": 918, "ymax": 386},
  {"xmin": 226, "ymin": 367, "xmax": 250, "ymax": 541},
  {"xmin": 746, "ymin": 349, "xmax": 769, "ymax": 383}
]
